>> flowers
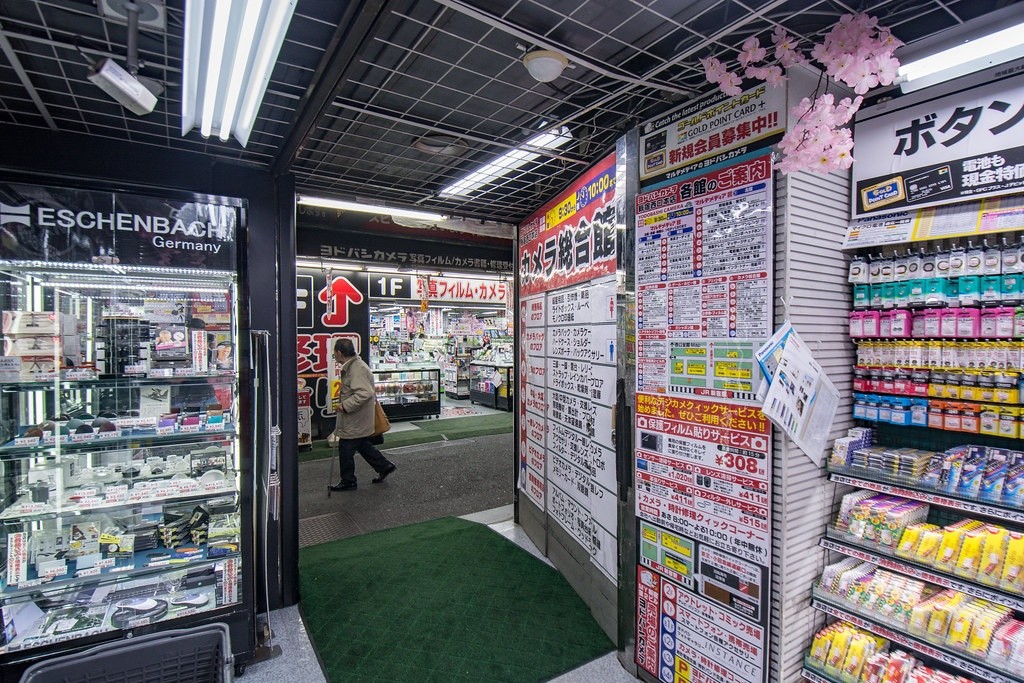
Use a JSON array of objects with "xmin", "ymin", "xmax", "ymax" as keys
[{"xmin": 699, "ymin": 14, "xmax": 908, "ymax": 176}]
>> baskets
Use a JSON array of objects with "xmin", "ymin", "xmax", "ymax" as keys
[{"xmin": 20, "ymin": 622, "xmax": 234, "ymax": 683}]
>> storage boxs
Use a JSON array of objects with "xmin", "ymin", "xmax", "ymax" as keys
[{"xmin": 19, "ymin": 622, "xmax": 234, "ymax": 683}]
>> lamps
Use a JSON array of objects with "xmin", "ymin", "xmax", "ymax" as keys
[
  {"xmin": 896, "ymin": 0, "xmax": 1024, "ymax": 94},
  {"xmin": 436, "ymin": 114, "xmax": 573, "ymax": 196},
  {"xmin": 181, "ymin": 0, "xmax": 298, "ymax": 149},
  {"xmin": 523, "ymin": 50, "xmax": 568, "ymax": 83}
]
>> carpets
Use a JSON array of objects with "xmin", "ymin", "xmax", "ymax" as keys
[{"xmin": 297, "ymin": 516, "xmax": 616, "ymax": 683}]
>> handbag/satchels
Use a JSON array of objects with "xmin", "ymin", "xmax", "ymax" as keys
[{"xmin": 371, "ymin": 401, "xmax": 391, "ymax": 436}]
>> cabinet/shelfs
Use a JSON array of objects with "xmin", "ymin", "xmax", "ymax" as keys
[
  {"xmin": 800, "ymin": 191, "xmax": 1024, "ymax": 683},
  {"xmin": 0, "ymin": 260, "xmax": 260, "ymax": 677},
  {"xmin": 368, "ymin": 302, "xmax": 514, "ymax": 419}
]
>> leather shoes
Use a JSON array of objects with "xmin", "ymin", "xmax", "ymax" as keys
[
  {"xmin": 371, "ymin": 462, "xmax": 397, "ymax": 484},
  {"xmin": 327, "ymin": 478, "xmax": 358, "ymax": 491}
]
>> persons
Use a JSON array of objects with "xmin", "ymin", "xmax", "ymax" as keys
[{"xmin": 327, "ymin": 338, "xmax": 397, "ymax": 492}]
